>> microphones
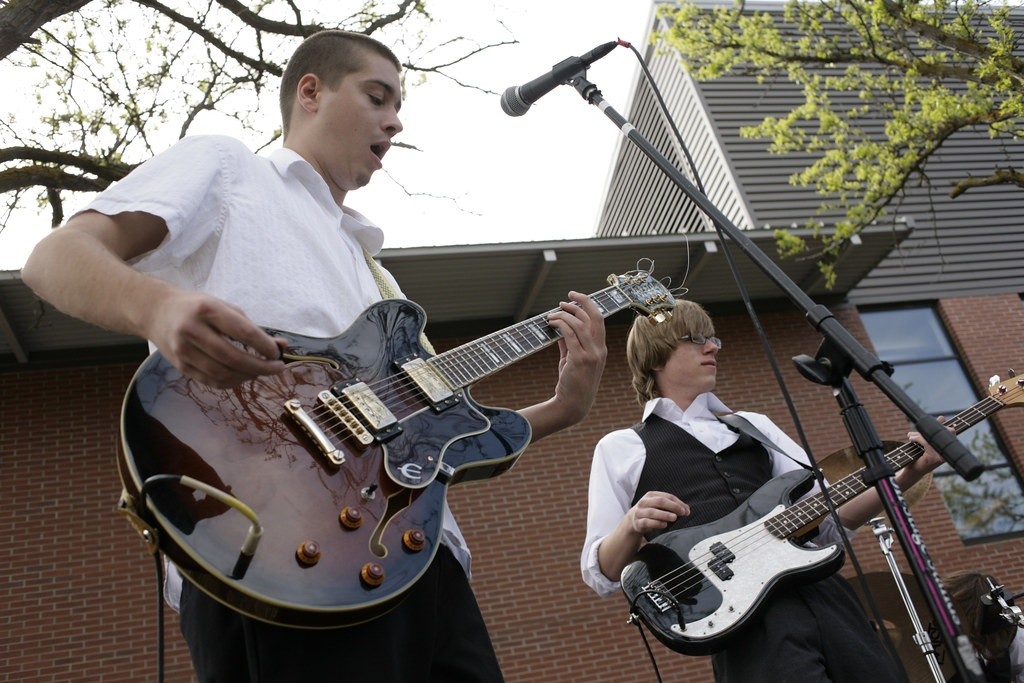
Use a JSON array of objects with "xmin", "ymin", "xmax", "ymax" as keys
[{"xmin": 501, "ymin": 42, "xmax": 616, "ymax": 117}]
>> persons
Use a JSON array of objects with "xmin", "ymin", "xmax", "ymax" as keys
[
  {"xmin": 581, "ymin": 299, "xmax": 958, "ymax": 683},
  {"xmin": 21, "ymin": 31, "xmax": 608, "ymax": 683},
  {"xmin": 942, "ymin": 570, "xmax": 1024, "ymax": 683}
]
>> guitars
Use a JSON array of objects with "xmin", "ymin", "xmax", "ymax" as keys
[
  {"xmin": 112, "ymin": 269, "xmax": 677, "ymax": 629},
  {"xmin": 620, "ymin": 366, "xmax": 1024, "ymax": 655}
]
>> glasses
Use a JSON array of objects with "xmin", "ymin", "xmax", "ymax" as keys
[{"xmin": 679, "ymin": 330, "xmax": 722, "ymax": 349}]
